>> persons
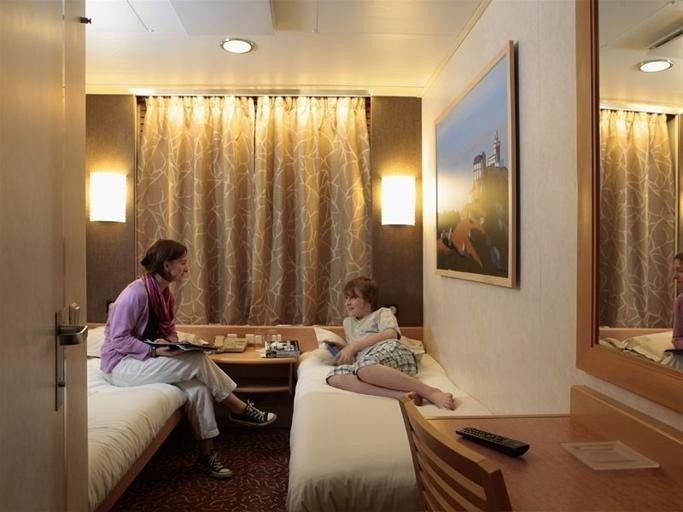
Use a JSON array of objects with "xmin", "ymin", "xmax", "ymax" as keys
[
  {"xmin": 97, "ymin": 237, "xmax": 278, "ymax": 478},
  {"xmin": 658, "ymin": 252, "xmax": 683, "ymax": 370},
  {"xmin": 325, "ymin": 275, "xmax": 456, "ymax": 411}
]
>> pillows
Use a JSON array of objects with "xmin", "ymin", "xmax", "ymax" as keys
[
  {"xmin": 623, "ymin": 331, "xmax": 675, "ymax": 362},
  {"xmin": 313, "ymin": 327, "xmax": 425, "ymax": 365},
  {"xmin": 87, "ymin": 326, "xmax": 209, "ymax": 358}
]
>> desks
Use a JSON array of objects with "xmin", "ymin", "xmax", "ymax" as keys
[{"xmin": 424, "ymin": 413, "xmax": 683, "ymax": 512}]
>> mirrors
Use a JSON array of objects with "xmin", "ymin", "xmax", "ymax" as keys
[{"xmin": 575, "ymin": 0, "xmax": 683, "ymax": 414}]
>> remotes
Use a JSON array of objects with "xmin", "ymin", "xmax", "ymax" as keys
[
  {"xmin": 327, "ymin": 342, "xmax": 339, "ymax": 356},
  {"xmin": 456, "ymin": 426, "xmax": 531, "ymax": 458}
]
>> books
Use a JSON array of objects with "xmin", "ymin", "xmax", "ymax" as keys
[{"xmin": 143, "ymin": 337, "xmax": 220, "ymax": 352}]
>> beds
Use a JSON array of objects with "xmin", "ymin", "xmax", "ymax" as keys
[
  {"xmin": 88, "ymin": 358, "xmax": 189, "ymax": 512},
  {"xmin": 287, "ymin": 351, "xmax": 492, "ymax": 512},
  {"xmin": 600, "ymin": 331, "xmax": 675, "ymax": 363}
]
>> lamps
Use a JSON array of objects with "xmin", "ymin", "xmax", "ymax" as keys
[
  {"xmin": 89, "ymin": 171, "xmax": 127, "ymax": 224},
  {"xmin": 220, "ymin": 38, "xmax": 254, "ymax": 54},
  {"xmin": 648, "ymin": 27, "xmax": 683, "ymax": 49},
  {"xmin": 639, "ymin": 59, "xmax": 673, "ymax": 73},
  {"xmin": 381, "ymin": 175, "xmax": 416, "ymax": 226}
]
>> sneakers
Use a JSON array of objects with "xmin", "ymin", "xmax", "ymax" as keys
[
  {"xmin": 197, "ymin": 448, "xmax": 232, "ymax": 477},
  {"xmin": 229, "ymin": 399, "xmax": 276, "ymax": 426}
]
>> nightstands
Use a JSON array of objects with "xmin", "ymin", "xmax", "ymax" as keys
[{"xmin": 208, "ymin": 347, "xmax": 297, "ymax": 412}]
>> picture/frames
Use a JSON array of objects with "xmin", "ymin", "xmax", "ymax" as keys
[{"xmin": 431, "ymin": 40, "xmax": 516, "ymax": 290}]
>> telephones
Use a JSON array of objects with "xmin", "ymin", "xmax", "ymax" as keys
[{"xmin": 214, "ymin": 335, "xmax": 249, "ymax": 353}]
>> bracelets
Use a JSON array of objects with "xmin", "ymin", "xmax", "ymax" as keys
[{"xmin": 151, "ymin": 345, "xmax": 156, "ymax": 357}]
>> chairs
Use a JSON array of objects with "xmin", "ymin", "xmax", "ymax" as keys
[{"xmin": 397, "ymin": 395, "xmax": 512, "ymax": 512}]
